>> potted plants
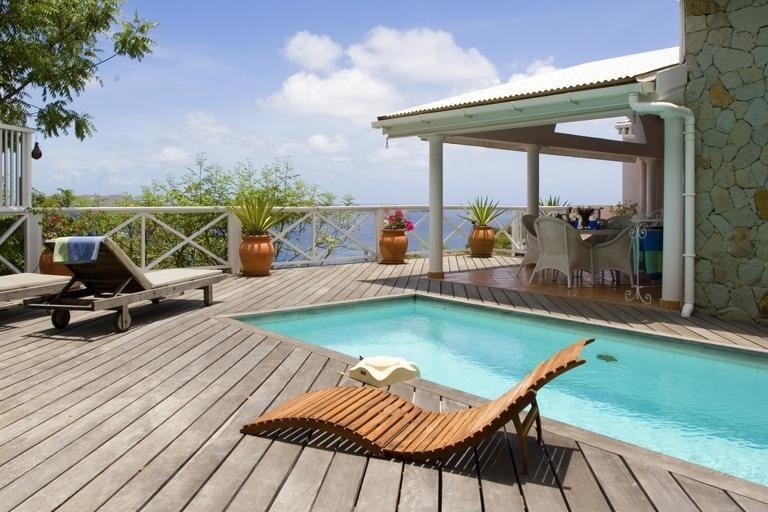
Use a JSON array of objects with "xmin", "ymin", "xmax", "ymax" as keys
[
  {"xmin": 457, "ymin": 193, "xmax": 510, "ymax": 257},
  {"xmin": 222, "ymin": 185, "xmax": 298, "ymax": 275}
]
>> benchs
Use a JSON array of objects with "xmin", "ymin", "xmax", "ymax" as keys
[{"xmin": 0, "ymin": 268, "xmax": 82, "ymax": 317}]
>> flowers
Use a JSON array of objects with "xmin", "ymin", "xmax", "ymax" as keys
[
  {"xmin": 383, "ymin": 207, "xmax": 414, "ymax": 231},
  {"xmin": 576, "ymin": 207, "xmax": 595, "ymax": 216},
  {"xmin": 38, "ymin": 214, "xmax": 84, "ymax": 241}
]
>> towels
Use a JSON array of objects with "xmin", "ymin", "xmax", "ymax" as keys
[{"xmin": 632, "ymin": 229, "xmax": 663, "ymax": 280}]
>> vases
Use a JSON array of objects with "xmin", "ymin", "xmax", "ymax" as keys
[
  {"xmin": 39, "ymin": 240, "xmax": 75, "ymax": 276},
  {"xmin": 379, "ymin": 228, "xmax": 408, "ymax": 263}
]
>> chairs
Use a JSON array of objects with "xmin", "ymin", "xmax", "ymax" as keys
[
  {"xmin": 238, "ymin": 337, "xmax": 596, "ymax": 475},
  {"xmin": 22, "ymin": 236, "xmax": 227, "ymax": 333},
  {"xmin": 515, "ymin": 214, "xmax": 636, "ymax": 289}
]
